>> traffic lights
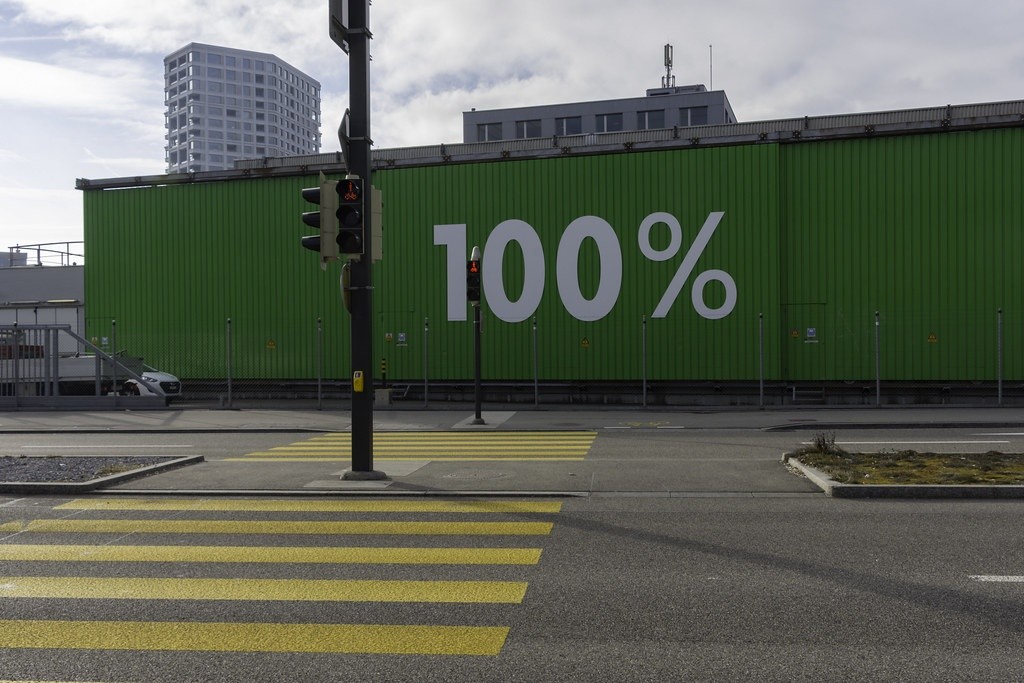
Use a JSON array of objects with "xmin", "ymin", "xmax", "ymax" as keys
[
  {"xmin": 334, "ymin": 177, "xmax": 366, "ymax": 256},
  {"xmin": 300, "ymin": 185, "xmax": 324, "ymax": 250},
  {"xmin": 466, "ymin": 257, "xmax": 483, "ymax": 301}
]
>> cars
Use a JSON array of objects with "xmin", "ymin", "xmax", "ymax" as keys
[{"xmin": 119, "ymin": 362, "xmax": 181, "ymax": 400}]
